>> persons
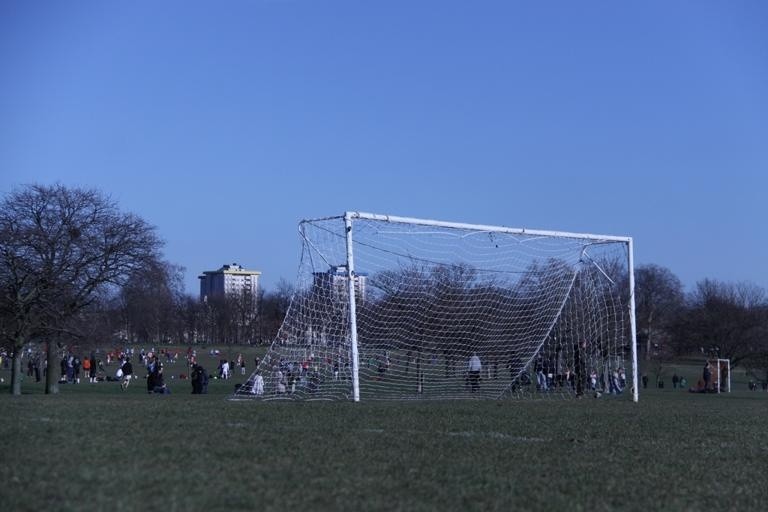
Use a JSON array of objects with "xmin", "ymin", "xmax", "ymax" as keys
[
  {"xmin": 376, "ymin": 350, "xmax": 626, "ymax": 398},
  {"xmin": 702, "ymin": 362, "xmax": 711, "ymax": 391},
  {"xmin": 748, "ymin": 380, "xmax": 754, "ymax": 389},
  {"xmin": 642, "ymin": 373, "xmax": 688, "ymax": 389},
  {"xmin": 2, "ymin": 345, "xmax": 353, "ymax": 395}
]
